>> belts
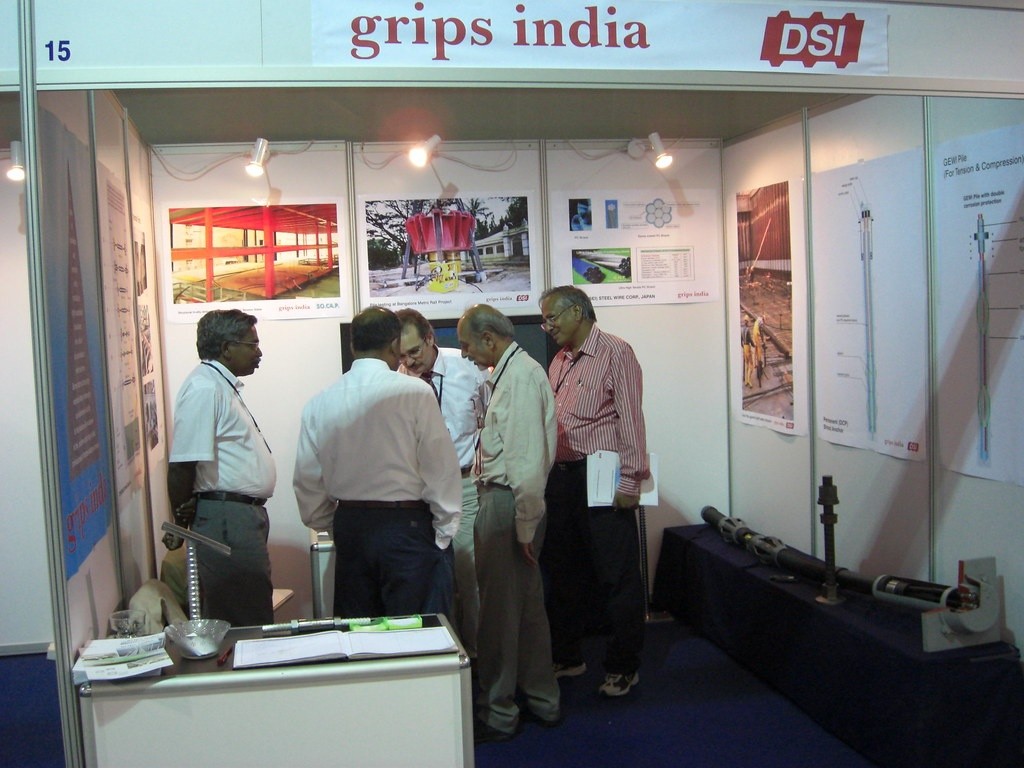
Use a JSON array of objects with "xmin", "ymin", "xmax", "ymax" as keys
[
  {"xmin": 461, "ymin": 464, "xmax": 474, "ymax": 475},
  {"xmin": 337, "ymin": 500, "xmax": 427, "ymax": 509},
  {"xmin": 196, "ymin": 490, "xmax": 267, "ymax": 507},
  {"xmin": 552, "ymin": 458, "xmax": 586, "ymax": 471}
]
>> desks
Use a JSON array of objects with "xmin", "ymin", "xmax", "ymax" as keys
[{"xmin": 79, "ymin": 612, "xmax": 473, "ymax": 768}]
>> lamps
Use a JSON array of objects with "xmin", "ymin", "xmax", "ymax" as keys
[
  {"xmin": 245, "ymin": 138, "xmax": 270, "ymax": 178},
  {"xmin": 627, "ymin": 132, "xmax": 673, "ymax": 168},
  {"xmin": 408, "ymin": 134, "xmax": 442, "ymax": 168}
]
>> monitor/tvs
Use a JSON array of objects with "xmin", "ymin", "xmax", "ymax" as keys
[{"xmin": 340, "ymin": 315, "xmax": 562, "ymax": 381}]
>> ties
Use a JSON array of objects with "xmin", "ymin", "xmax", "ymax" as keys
[{"xmin": 421, "ymin": 371, "xmax": 438, "ymax": 399}]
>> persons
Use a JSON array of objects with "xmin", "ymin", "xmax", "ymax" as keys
[
  {"xmin": 393, "ymin": 309, "xmax": 491, "ymax": 680},
  {"xmin": 292, "ymin": 306, "xmax": 463, "ymax": 621},
  {"xmin": 540, "ymin": 286, "xmax": 649, "ymax": 696},
  {"xmin": 163, "ymin": 309, "xmax": 277, "ymax": 626},
  {"xmin": 753, "ymin": 315, "xmax": 766, "ymax": 368},
  {"xmin": 739, "ymin": 315, "xmax": 756, "ymax": 390},
  {"xmin": 457, "ymin": 303, "xmax": 560, "ymax": 743}
]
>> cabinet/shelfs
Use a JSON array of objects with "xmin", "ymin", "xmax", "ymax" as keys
[{"xmin": 653, "ymin": 523, "xmax": 1024, "ymax": 768}]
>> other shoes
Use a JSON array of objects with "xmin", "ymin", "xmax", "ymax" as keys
[
  {"xmin": 519, "ymin": 705, "xmax": 557, "ymax": 729},
  {"xmin": 474, "ymin": 717, "xmax": 511, "ymax": 744}
]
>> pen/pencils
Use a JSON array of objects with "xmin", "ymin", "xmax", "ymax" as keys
[
  {"xmin": 578, "ymin": 379, "xmax": 581, "ymax": 386},
  {"xmin": 218, "ymin": 646, "xmax": 232, "ymax": 664},
  {"xmin": 470, "ymin": 399, "xmax": 476, "ymax": 410}
]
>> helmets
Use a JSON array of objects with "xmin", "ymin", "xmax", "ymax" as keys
[{"xmin": 745, "ymin": 315, "xmax": 749, "ymax": 322}]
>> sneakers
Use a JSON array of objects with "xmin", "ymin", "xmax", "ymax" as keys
[
  {"xmin": 599, "ymin": 669, "xmax": 639, "ymax": 697},
  {"xmin": 552, "ymin": 657, "xmax": 587, "ymax": 677}
]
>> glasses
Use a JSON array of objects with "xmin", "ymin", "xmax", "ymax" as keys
[
  {"xmin": 228, "ymin": 339, "xmax": 259, "ymax": 351},
  {"xmin": 540, "ymin": 303, "xmax": 575, "ymax": 331},
  {"xmin": 398, "ymin": 338, "xmax": 426, "ymax": 364}
]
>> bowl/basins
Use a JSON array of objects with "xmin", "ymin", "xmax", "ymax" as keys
[
  {"xmin": 109, "ymin": 610, "xmax": 146, "ymax": 638},
  {"xmin": 164, "ymin": 619, "xmax": 231, "ymax": 659}
]
